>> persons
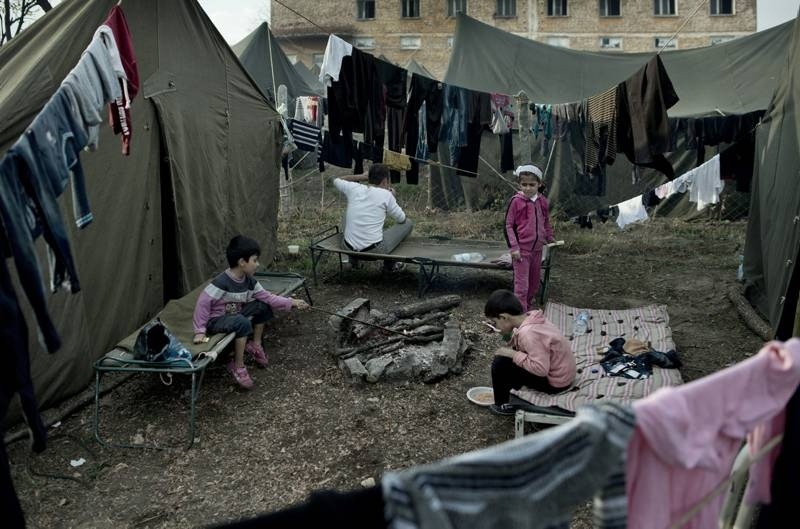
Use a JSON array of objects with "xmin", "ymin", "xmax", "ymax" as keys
[
  {"xmin": 505, "ymin": 164, "xmax": 554, "ymax": 314},
  {"xmin": 333, "ymin": 163, "xmax": 414, "ymax": 259},
  {"xmin": 483, "ymin": 290, "xmax": 577, "ymax": 415},
  {"xmin": 192, "ymin": 236, "xmax": 310, "ymax": 389}
]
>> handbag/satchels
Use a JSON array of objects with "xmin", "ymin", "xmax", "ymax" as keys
[{"xmin": 133, "ymin": 317, "xmax": 192, "ymax": 363}]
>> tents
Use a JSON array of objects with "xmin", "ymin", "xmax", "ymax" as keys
[
  {"xmin": 230, "ymin": 20, "xmax": 441, "ymax": 170},
  {"xmin": 432, "ymin": 10, "xmax": 800, "ymax": 210},
  {"xmin": 743, "ymin": 10, "xmax": 800, "ymax": 339},
  {"xmin": 0, "ymin": 1, "xmax": 280, "ymax": 445}
]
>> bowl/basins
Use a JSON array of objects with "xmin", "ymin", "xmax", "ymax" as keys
[{"xmin": 288, "ymin": 245, "xmax": 299, "ymax": 254}]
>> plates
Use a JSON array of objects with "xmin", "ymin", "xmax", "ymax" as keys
[{"xmin": 467, "ymin": 386, "xmax": 495, "ymax": 406}]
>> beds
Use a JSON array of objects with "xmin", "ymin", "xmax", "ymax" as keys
[
  {"xmin": 95, "ymin": 269, "xmax": 312, "ymax": 450},
  {"xmin": 310, "ymin": 225, "xmax": 555, "ymax": 306},
  {"xmin": 514, "ymin": 301, "xmax": 685, "ymax": 441}
]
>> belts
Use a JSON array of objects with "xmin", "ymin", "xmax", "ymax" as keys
[{"xmin": 344, "ymin": 239, "xmax": 381, "ymax": 253}]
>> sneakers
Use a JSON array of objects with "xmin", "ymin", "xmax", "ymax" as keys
[
  {"xmin": 245, "ymin": 340, "xmax": 268, "ymax": 364},
  {"xmin": 491, "ymin": 403, "xmax": 516, "ymax": 415},
  {"xmin": 226, "ymin": 359, "xmax": 254, "ymax": 389}
]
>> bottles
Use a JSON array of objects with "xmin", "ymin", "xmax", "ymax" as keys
[
  {"xmin": 572, "ymin": 310, "xmax": 590, "ymax": 335},
  {"xmin": 450, "ymin": 252, "xmax": 487, "ymax": 263}
]
